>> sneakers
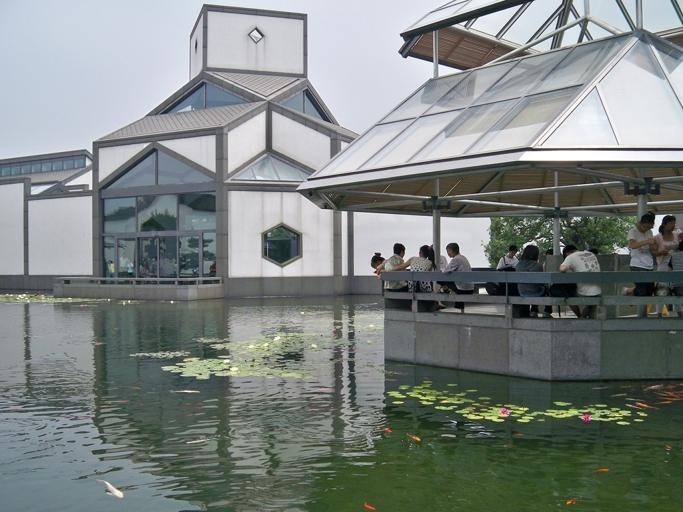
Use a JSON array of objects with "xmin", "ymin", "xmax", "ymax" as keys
[
  {"xmin": 543, "ymin": 312, "xmax": 553, "ymax": 318},
  {"xmin": 529, "ymin": 311, "xmax": 538, "ymax": 318}
]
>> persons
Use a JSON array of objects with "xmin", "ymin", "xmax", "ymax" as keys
[
  {"xmin": 374, "ymin": 242, "xmax": 409, "ymax": 292},
  {"xmin": 661, "ymin": 237, "xmax": 682, "ymax": 298},
  {"xmin": 432, "ymin": 242, "xmax": 474, "ymax": 313},
  {"xmin": 427, "ymin": 244, "xmax": 449, "ymax": 272},
  {"xmin": 650, "ymin": 214, "xmax": 683, "ymax": 319},
  {"xmin": 619, "ymin": 213, "xmax": 658, "ymax": 297},
  {"xmin": 514, "ymin": 244, "xmax": 554, "ymax": 319},
  {"xmin": 556, "ymin": 244, "xmax": 602, "ymax": 318},
  {"xmin": 386, "ymin": 245, "xmax": 446, "ymax": 312},
  {"xmin": 495, "ymin": 244, "xmax": 519, "ymax": 273},
  {"xmin": 369, "ymin": 252, "xmax": 386, "ymax": 268},
  {"xmin": 104, "ymin": 255, "xmax": 215, "ymax": 282},
  {"xmin": 541, "ymin": 248, "xmax": 554, "ymax": 272}
]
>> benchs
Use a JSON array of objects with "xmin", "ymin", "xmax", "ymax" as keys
[{"xmin": 384, "ymin": 266, "xmax": 682, "ymax": 319}]
